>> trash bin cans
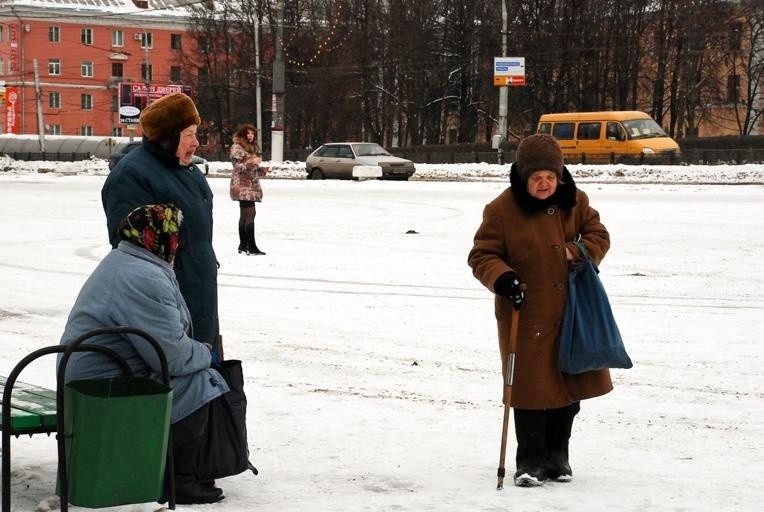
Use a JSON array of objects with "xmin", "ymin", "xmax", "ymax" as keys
[{"xmin": 54, "ymin": 375, "xmax": 174, "ymax": 509}]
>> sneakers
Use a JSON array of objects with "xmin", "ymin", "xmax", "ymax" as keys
[
  {"xmin": 514, "ymin": 463, "xmax": 545, "ymax": 486},
  {"xmin": 548, "ymin": 458, "xmax": 573, "ymax": 481}
]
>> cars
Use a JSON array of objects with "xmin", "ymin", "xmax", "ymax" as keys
[{"xmin": 306, "ymin": 141, "xmax": 416, "ymax": 183}]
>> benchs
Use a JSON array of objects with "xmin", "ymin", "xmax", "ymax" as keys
[{"xmin": 0, "ymin": 344, "xmax": 135, "ymax": 511}]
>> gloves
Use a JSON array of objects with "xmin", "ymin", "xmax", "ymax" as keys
[{"xmin": 499, "ymin": 277, "xmax": 526, "ymax": 311}]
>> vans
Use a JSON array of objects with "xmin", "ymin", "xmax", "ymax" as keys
[{"xmin": 538, "ymin": 111, "xmax": 681, "ymax": 165}]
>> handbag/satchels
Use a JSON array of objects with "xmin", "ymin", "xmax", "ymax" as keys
[
  {"xmin": 183, "ymin": 359, "xmax": 249, "ymax": 480},
  {"xmin": 552, "ymin": 242, "xmax": 635, "ymax": 375}
]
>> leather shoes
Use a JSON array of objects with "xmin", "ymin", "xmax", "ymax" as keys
[{"xmin": 172, "ymin": 479, "xmax": 226, "ymax": 505}]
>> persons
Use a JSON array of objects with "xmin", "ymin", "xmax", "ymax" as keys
[
  {"xmin": 102, "ymin": 94, "xmax": 219, "ymax": 359},
  {"xmin": 230, "ymin": 125, "xmax": 270, "ymax": 255},
  {"xmin": 467, "ymin": 133, "xmax": 610, "ymax": 488},
  {"xmin": 57, "ymin": 205, "xmax": 229, "ymax": 506}
]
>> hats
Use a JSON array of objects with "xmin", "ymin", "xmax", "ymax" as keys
[
  {"xmin": 139, "ymin": 92, "xmax": 201, "ymax": 139},
  {"xmin": 515, "ymin": 134, "xmax": 563, "ymax": 177}
]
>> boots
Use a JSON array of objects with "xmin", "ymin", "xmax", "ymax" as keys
[
  {"xmin": 237, "ymin": 225, "xmax": 247, "ymax": 253},
  {"xmin": 245, "ymin": 224, "xmax": 266, "ymax": 256}
]
